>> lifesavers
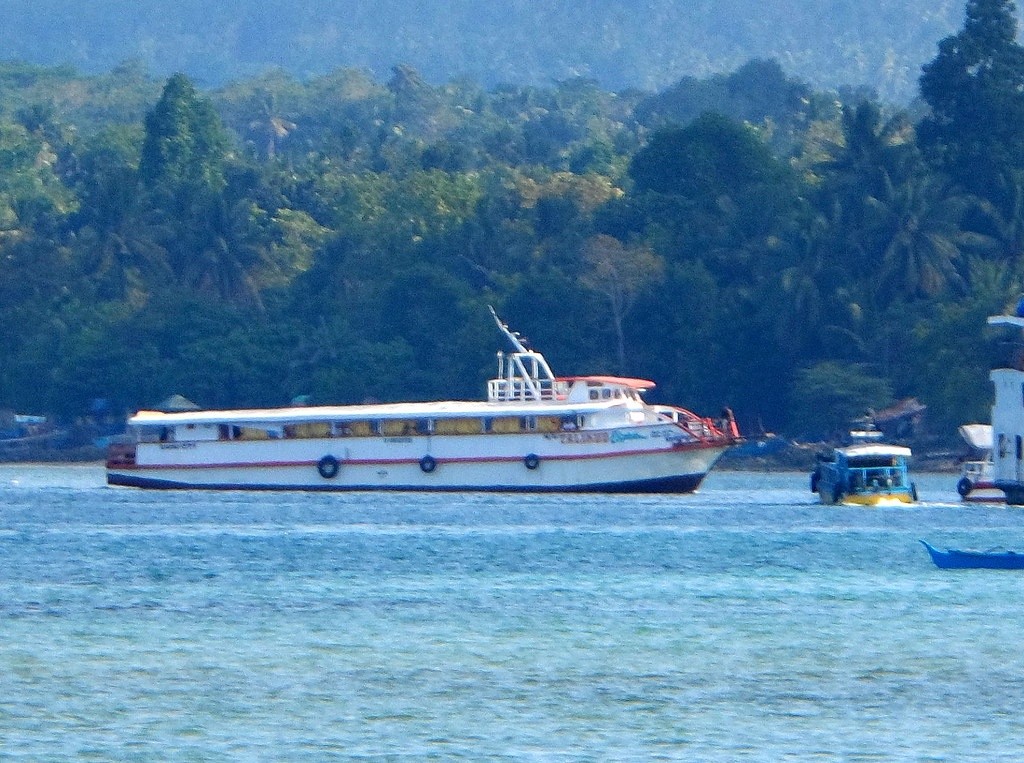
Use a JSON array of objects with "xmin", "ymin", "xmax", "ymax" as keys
[
  {"xmin": 524, "ymin": 454, "xmax": 539, "ymax": 469},
  {"xmin": 420, "ymin": 456, "xmax": 435, "ymax": 472},
  {"xmin": 956, "ymin": 478, "xmax": 972, "ymax": 495},
  {"xmin": 317, "ymin": 455, "xmax": 339, "ymax": 479}
]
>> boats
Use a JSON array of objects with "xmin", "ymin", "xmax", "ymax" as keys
[
  {"xmin": 956, "ymin": 293, "xmax": 1024, "ymax": 507},
  {"xmin": 918, "ymin": 538, "xmax": 1024, "ymax": 569},
  {"xmin": 105, "ymin": 304, "xmax": 775, "ymax": 493},
  {"xmin": 810, "ymin": 408, "xmax": 919, "ymax": 505}
]
